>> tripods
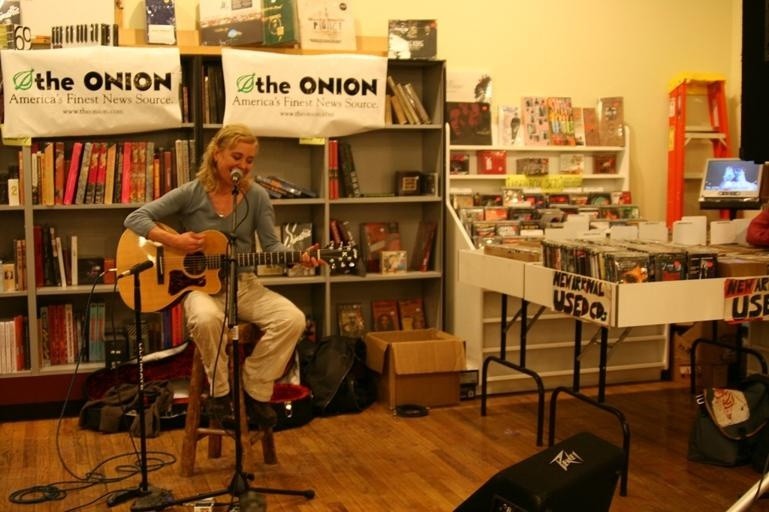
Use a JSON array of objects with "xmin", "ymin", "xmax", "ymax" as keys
[{"xmin": 162, "ymin": 182, "xmax": 315, "ymax": 512}]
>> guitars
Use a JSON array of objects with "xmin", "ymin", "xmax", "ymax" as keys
[{"xmin": 116, "ymin": 222, "xmax": 358, "ymax": 313}]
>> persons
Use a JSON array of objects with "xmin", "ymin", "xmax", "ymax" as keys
[{"xmin": 124, "ymin": 123, "xmax": 327, "ymax": 429}]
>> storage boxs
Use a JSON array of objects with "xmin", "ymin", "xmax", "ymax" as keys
[{"xmin": 363, "ymin": 326, "xmax": 468, "ymax": 412}]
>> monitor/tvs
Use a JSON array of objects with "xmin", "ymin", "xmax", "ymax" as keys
[{"xmin": 698, "ymin": 158, "xmax": 764, "ymax": 202}]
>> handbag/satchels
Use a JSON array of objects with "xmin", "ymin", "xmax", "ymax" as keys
[{"xmin": 685, "ymin": 374, "xmax": 768, "ymax": 468}]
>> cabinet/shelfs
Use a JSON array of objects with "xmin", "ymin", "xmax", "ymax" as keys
[
  {"xmin": 199, "ymin": 44, "xmax": 449, "ymax": 358},
  {"xmin": 2, "ymin": 44, "xmax": 199, "ymax": 416},
  {"xmin": 438, "ymin": 124, "xmax": 677, "ymax": 398}
]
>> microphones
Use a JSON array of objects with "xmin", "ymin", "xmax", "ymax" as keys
[
  {"xmin": 117, "ymin": 260, "xmax": 154, "ymax": 278},
  {"xmin": 230, "ymin": 167, "xmax": 243, "ymax": 183}
]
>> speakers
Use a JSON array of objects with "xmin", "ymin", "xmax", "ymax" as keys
[
  {"xmin": 301, "ymin": 335, "xmax": 375, "ymax": 415},
  {"xmin": 451, "ymin": 430, "xmax": 626, "ymax": 512}
]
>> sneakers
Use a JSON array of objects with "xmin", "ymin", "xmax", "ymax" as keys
[
  {"xmin": 209, "ymin": 383, "xmax": 232, "ymax": 415},
  {"xmin": 248, "ymin": 397, "xmax": 276, "ymax": 425}
]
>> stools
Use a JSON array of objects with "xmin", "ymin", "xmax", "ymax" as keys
[{"xmin": 177, "ymin": 320, "xmax": 278, "ymax": 477}]
